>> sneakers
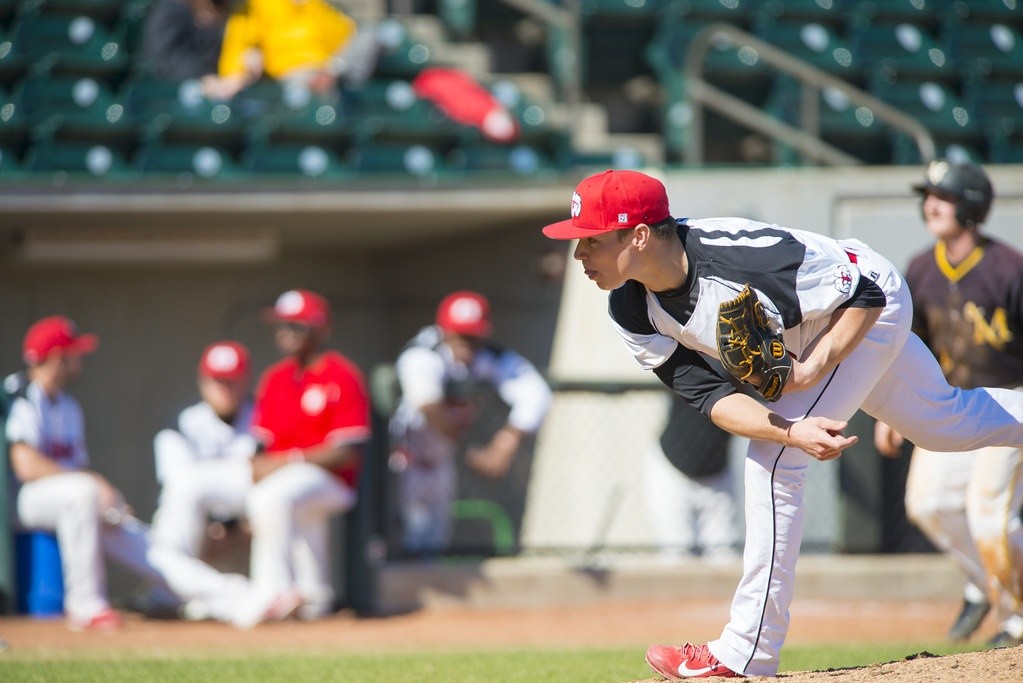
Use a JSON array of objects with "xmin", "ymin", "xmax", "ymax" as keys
[{"xmin": 645, "ymin": 641, "xmax": 744, "ymax": 682}]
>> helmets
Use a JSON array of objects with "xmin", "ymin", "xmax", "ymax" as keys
[{"xmin": 913, "ymin": 160, "xmax": 994, "ymax": 224}]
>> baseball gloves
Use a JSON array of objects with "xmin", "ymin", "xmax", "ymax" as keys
[{"xmin": 715, "ymin": 282, "xmax": 793, "ymax": 401}]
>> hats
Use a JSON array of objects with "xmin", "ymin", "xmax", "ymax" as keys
[
  {"xmin": 275, "ymin": 288, "xmax": 327, "ymax": 328},
  {"xmin": 24, "ymin": 316, "xmax": 95, "ymax": 363},
  {"xmin": 435, "ymin": 289, "xmax": 493, "ymax": 337},
  {"xmin": 201, "ymin": 343, "xmax": 250, "ymax": 383},
  {"xmin": 542, "ymin": 169, "xmax": 670, "ymax": 240}
]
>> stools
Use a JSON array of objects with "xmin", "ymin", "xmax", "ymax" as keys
[{"xmin": 11, "ymin": 529, "xmax": 64, "ymax": 616}]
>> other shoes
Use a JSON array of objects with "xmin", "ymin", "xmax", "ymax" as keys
[
  {"xmin": 68, "ymin": 610, "xmax": 122, "ymax": 633},
  {"xmin": 992, "ymin": 614, "xmax": 1023, "ymax": 649},
  {"xmin": 949, "ymin": 599, "xmax": 991, "ymax": 640},
  {"xmin": 265, "ymin": 598, "xmax": 301, "ymax": 620}
]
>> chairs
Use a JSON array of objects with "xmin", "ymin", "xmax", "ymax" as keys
[{"xmin": 0, "ymin": 0, "xmax": 1023, "ymax": 174}]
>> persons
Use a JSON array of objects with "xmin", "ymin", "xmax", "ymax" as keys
[
  {"xmin": 129, "ymin": 0, "xmax": 228, "ymax": 118},
  {"xmin": 2, "ymin": 316, "xmax": 266, "ymax": 630},
  {"xmin": 543, "ymin": 171, "xmax": 1023, "ymax": 681},
  {"xmin": 251, "ymin": 291, "xmax": 376, "ymax": 616},
  {"xmin": 874, "ymin": 155, "xmax": 1023, "ymax": 650},
  {"xmin": 218, "ymin": 0, "xmax": 355, "ymax": 83},
  {"xmin": 657, "ymin": 404, "xmax": 745, "ymax": 557},
  {"xmin": 377, "ymin": 291, "xmax": 551, "ymax": 553},
  {"xmin": 153, "ymin": 339, "xmax": 253, "ymax": 558}
]
